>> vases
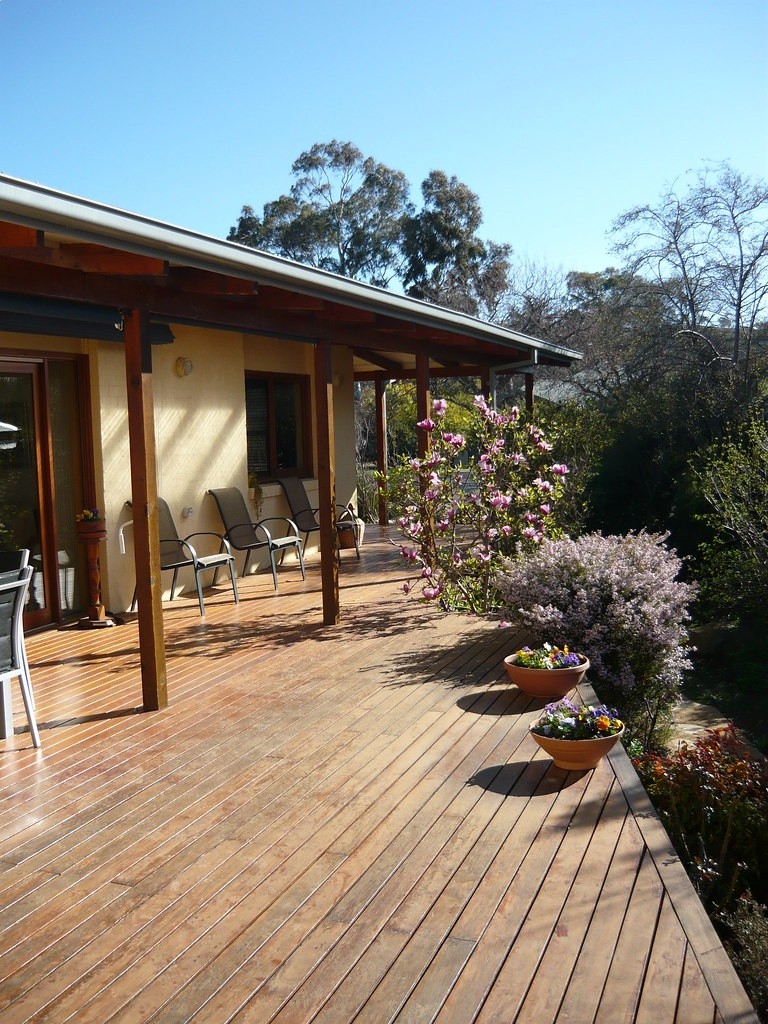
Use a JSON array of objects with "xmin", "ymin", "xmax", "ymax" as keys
[
  {"xmin": 504, "ymin": 651, "xmax": 589, "ymax": 698},
  {"xmin": 77, "ymin": 518, "xmax": 106, "ymax": 533},
  {"xmin": 528, "ymin": 718, "xmax": 624, "ymax": 769}
]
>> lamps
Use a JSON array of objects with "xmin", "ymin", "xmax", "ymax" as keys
[{"xmin": 176, "ymin": 357, "xmax": 193, "ymax": 377}]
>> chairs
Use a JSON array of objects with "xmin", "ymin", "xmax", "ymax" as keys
[
  {"xmin": 0, "ymin": 549, "xmax": 41, "ymax": 749},
  {"xmin": 206, "ymin": 486, "xmax": 305, "ymax": 590},
  {"xmin": 125, "ymin": 497, "xmax": 238, "ymax": 616},
  {"xmin": 277, "ymin": 476, "xmax": 360, "ymax": 566}
]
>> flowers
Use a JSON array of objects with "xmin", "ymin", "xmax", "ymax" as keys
[
  {"xmin": 75, "ymin": 508, "xmax": 103, "ymax": 522},
  {"xmin": 533, "ymin": 697, "xmax": 621, "ymax": 738},
  {"xmin": 513, "ymin": 642, "xmax": 578, "ymax": 668}
]
